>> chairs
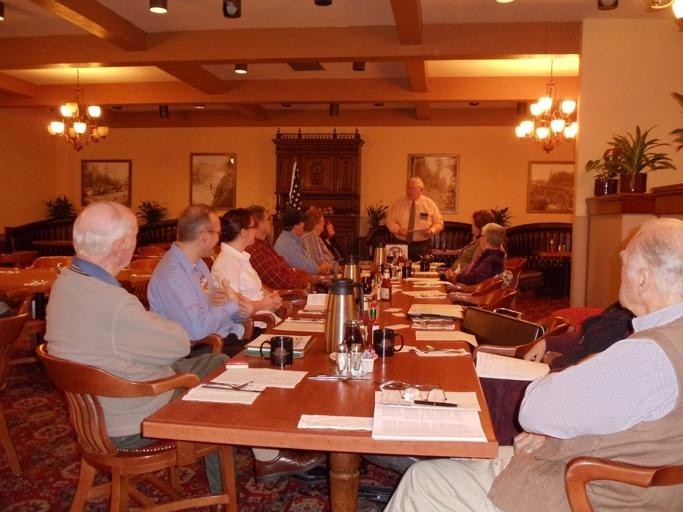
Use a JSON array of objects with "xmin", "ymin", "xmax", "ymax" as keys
[
  {"xmin": 446, "ymin": 246, "xmax": 683, "ymax": 512},
  {"xmin": 2, "ymin": 246, "xmax": 310, "ymax": 351},
  {"xmin": 36, "ymin": 345, "xmax": 239, "ymax": 512},
  {"xmin": 2, "ymin": 312, "xmax": 32, "ymax": 477}
]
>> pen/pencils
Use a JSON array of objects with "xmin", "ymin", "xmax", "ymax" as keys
[{"xmin": 415, "ymin": 400, "xmax": 457, "ymax": 407}]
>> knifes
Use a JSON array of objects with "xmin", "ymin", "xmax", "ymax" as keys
[{"xmin": 202, "ymin": 386, "xmax": 262, "ymax": 393}]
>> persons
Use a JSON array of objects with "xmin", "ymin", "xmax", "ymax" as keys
[
  {"xmin": 379, "ymin": 217, "xmax": 683, "ymax": 512},
  {"xmin": 360, "ymin": 299, "xmax": 637, "ymax": 477},
  {"xmin": 208, "ymin": 207, "xmax": 284, "ymax": 332},
  {"xmin": 147, "ymin": 204, "xmax": 329, "ymax": 485},
  {"xmin": 439, "ymin": 222, "xmax": 506, "ymax": 287},
  {"xmin": 301, "ymin": 206, "xmax": 337, "ymax": 270},
  {"xmin": 272, "ymin": 207, "xmax": 319, "ymax": 276},
  {"xmin": 245, "ymin": 204, "xmax": 322, "ymax": 292},
  {"xmin": 42, "ymin": 200, "xmax": 326, "ymax": 485},
  {"xmin": 450, "ymin": 208, "xmax": 496, "ymax": 269},
  {"xmin": 386, "ymin": 177, "xmax": 445, "ymax": 265},
  {"xmin": 320, "ymin": 218, "xmax": 345, "ymax": 267}
]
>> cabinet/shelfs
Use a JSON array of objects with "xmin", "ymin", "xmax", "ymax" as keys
[{"xmin": 272, "ymin": 128, "xmax": 365, "ymax": 264}]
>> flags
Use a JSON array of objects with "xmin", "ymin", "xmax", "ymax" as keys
[{"xmin": 288, "ymin": 156, "xmax": 303, "ymax": 211}]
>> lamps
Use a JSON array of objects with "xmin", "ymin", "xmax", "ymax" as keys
[
  {"xmin": 47, "ymin": 68, "xmax": 110, "ymax": 151},
  {"xmin": 515, "ymin": 58, "xmax": 580, "ymax": 152},
  {"xmin": 650, "ymin": 1, "xmax": 683, "ymax": 32},
  {"xmin": 149, "ymin": 0, "xmax": 365, "ymax": 118}
]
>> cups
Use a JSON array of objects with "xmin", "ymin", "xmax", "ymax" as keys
[
  {"xmin": 311, "ymin": 282, "xmax": 328, "ymax": 294},
  {"xmin": 374, "ymin": 328, "xmax": 404, "ymax": 356},
  {"xmin": 420, "ymin": 262, "xmax": 430, "ymax": 272},
  {"xmin": 402, "ymin": 266, "xmax": 415, "ymax": 277},
  {"xmin": 260, "ymin": 336, "xmax": 293, "ymax": 366}
]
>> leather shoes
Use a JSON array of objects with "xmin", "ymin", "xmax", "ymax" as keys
[
  {"xmin": 289, "ymin": 467, "xmax": 328, "ymax": 483},
  {"xmin": 253, "ymin": 449, "xmax": 327, "ymax": 485}
]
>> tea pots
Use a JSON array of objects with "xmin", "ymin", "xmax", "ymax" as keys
[
  {"xmin": 324, "ymin": 255, "xmax": 362, "ymax": 353},
  {"xmin": 374, "ymin": 245, "xmax": 385, "ymax": 266}
]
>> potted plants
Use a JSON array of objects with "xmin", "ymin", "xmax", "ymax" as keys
[{"xmin": 585, "ymin": 124, "xmax": 677, "ymax": 196}]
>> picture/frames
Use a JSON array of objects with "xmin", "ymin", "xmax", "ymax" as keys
[
  {"xmin": 81, "ymin": 159, "xmax": 132, "ymax": 208},
  {"xmin": 407, "ymin": 153, "xmax": 460, "ymax": 214},
  {"xmin": 189, "ymin": 152, "xmax": 237, "ymax": 211},
  {"xmin": 526, "ymin": 161, "xmax": 575, "ymax": 214}
]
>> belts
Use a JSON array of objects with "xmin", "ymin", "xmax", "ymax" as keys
[
  {"xmin": 396, "ymin": 238, "xmax": 431, "ymax": 244},
  {"xmin": 191, "ymin": 342, "xmax": 208, "ymax": 350}
]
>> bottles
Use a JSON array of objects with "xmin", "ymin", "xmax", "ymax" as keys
[
  {"xmin": 336, "ymin": 294, "xmax": 379, "ymax": 378},
  {"xmin": 388, "ymin": 249, "xmax": 405, "ymax": 265},
  {"xmin": 373, "ymin": 267, "xmax": 391, "ymax": 302}
]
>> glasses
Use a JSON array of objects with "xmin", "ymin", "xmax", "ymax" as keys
[{"xmin": 396, "ymin": 374, "xmax": 448, "ymax": 403}]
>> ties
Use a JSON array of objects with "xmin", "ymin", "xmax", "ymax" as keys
[{"xmin": 406, "ymin": 201, "xmax": 416, "ymax": 244}]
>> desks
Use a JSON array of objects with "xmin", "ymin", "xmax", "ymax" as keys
[{"xmin": 141, "ymin": 261, "xmax": 500, "ymax": 511}]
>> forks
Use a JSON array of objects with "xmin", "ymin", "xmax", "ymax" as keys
[{"xmin": 206, "ymin": 379, "xmax": 255, "ymax": 390}]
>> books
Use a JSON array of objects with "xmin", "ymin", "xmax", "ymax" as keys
[
  {"xmin": 244, "ymin": 334, "xmax": 312, "ymax": 358},
  {"xmin": 370, "ymin": 388, "xmax": 488, "ymax": 443}
]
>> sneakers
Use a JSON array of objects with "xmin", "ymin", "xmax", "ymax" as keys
[{"xmin": 363, "ymin": 455, "xmax": 418, "ymax": 474}]
